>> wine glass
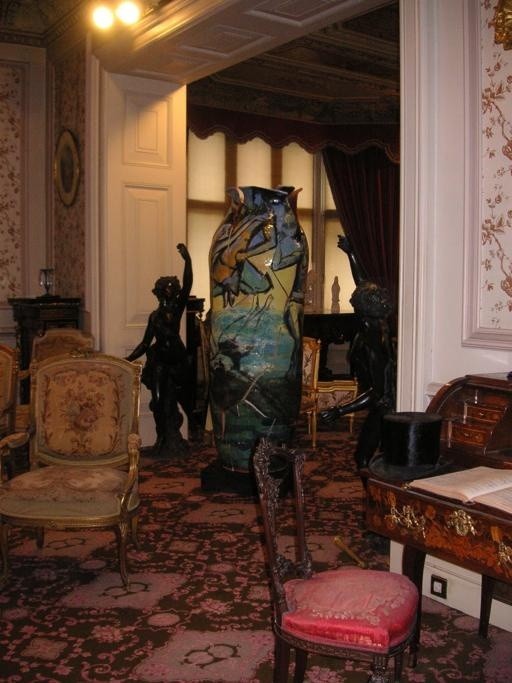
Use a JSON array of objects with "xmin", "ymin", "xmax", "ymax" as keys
[{"xmin": 35, "ymin": 268, "xmax": 59, "ymax": 297}]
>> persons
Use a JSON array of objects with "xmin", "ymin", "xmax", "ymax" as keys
[
  {"xmin": 128, "ymin": 243, "xmax": 192, "ymax": 447},
  {"xmin": 319, "ymin": 235, "xmax": 401, "ymax": 489}
]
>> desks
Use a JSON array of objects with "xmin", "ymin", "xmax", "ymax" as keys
[{"xmin": 299, "ymin": 313, "xmax": 361, "ymax": 379}]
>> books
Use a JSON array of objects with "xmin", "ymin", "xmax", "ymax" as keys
[{"xmin": 406, "ymin": 466, "xmax": 512, "ymax": 517}]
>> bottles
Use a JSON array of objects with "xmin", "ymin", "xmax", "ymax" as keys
[{"xmin": 330, "ymin": 275, "xmax": 340, "ymax": 302}]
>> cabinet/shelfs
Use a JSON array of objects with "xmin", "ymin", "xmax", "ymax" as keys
[{"xmin": 7, "ymin": 295, "xmax": 83, "ymax": 404}]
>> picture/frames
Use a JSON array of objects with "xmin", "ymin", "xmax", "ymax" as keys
[
  {"xmin": 53, "ymin": 129, "xmax": 82, "ymax": 207},
  {"xmin": 460, "ymin": 0, "xmax": 511, "ymax": 353}
]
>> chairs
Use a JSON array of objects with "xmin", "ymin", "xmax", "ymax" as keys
[
  {"xmin": 246, "ymin": 436, "xmax": 423, "ymax": 683},
  {"xmin": 299, "ymin": 332, "xmax": 360, "ymax": 452},
  {"xmin": 1, "ymin": 348, "xmax": 147, "ymax": 592},
  {"xmin": 16, "ymin": 326, "xmax": 96, "ymax": 432},
  {"xmin": 0, "ymin": 341, "xmax": 20, "ymax": 438}
]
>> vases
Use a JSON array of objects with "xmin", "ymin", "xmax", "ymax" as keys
[{"xmin": 196, "ymin": 183, "xmax": 311, "ymax": 505}]
